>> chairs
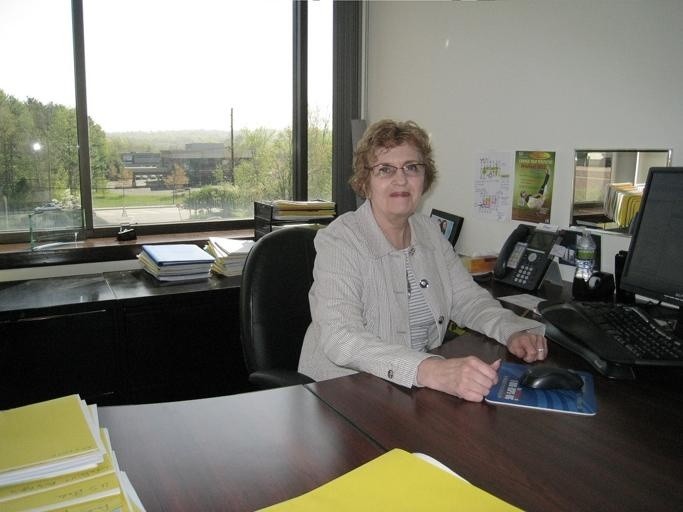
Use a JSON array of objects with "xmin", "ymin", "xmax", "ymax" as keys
[{"xmin": 239, "ymin": 227, "xmax": 316, "ymax": 389}]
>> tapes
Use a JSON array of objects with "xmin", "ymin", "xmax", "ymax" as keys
[{"xmin": 491, "ymin": 222, "xmax": 560, "ymax": 294}]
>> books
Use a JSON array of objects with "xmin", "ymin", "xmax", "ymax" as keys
[
  {"xmin": 137, "ymin": 237, "xmax": 257, "ymax": 283},
  {"xmin": 0, "ymin": 393, "xmax": 150, "ymax": 512},
  {"xmin": 263, "ymin": 198, "xmax": 337, "ymax": 229},
  {"xmin": 252, "ymin": 447, "xmax": 523, "ymax": 512},
  {"xmin": 603, "ymin": 182, "xmax": 646, "ymax": 230}
]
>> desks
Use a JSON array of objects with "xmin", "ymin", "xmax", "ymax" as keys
[
  {"xmin": 99, "ymin": 383, "xmax": 389, "ymax": 511},
  {"xmin": 300, "ymin": 280, "xmax": 683, "ymax": 510}
]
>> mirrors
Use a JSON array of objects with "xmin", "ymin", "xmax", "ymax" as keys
[{"xmin": 570, "ymin": 148, "xmax": 672, "ymax": 237}]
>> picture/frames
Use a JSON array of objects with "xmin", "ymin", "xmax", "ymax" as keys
[{"xmin": 431, "ymin": 209, "xmax": 463, "ymax": 246}]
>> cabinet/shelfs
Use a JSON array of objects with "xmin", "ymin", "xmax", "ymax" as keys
[{"xmin": 1, "ymin": 258, "xmax": 241, "ymax": 404}]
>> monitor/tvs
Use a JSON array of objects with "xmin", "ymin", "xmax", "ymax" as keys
[{"xmin": 620, "ymin": 166, "xmax": 683, "ymax": 344}]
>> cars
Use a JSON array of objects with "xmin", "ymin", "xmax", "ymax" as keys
[{"xmin": 34, "ymin": 202, "xmax": 63, "ymax": 214}]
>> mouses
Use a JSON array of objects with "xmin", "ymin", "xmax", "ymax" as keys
[{"xmin": 519, "ymin": 367, "xmax": 584, "ymax": 390}]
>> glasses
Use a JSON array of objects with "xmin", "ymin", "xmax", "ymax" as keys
[{"xmin": 365, "ymin": 161, "xmax": 427, "ymax": 178}]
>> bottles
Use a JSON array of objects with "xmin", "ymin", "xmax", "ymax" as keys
[{"xmin": 573, "ymin": 230, "xmax": 596, "ymax": 282}]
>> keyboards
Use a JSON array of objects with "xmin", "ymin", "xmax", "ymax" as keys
[{"xmin": 538, "ymin": 298, "xmax": 683, "ymax": 380}]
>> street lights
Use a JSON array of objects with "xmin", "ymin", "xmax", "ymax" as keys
[{"xmin": 32, "ymin": 140, "xmax": 52, "ymax": 201}]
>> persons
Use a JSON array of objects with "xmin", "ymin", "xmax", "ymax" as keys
[
  {"xmin": 517, "ymin": 168, "xmax": 551, "ymax": 212},
  {"xmin": 298, "ymin": 117, "xmax": 548, "ymax": 404},
  {"xmin": 438, "ymin": 219, "xmax": 447, "ymax": 236}
]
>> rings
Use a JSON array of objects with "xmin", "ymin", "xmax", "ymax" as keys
[{"xmin": 536, "ymin": 348, "xmax": 544, "ymax": 352}]
